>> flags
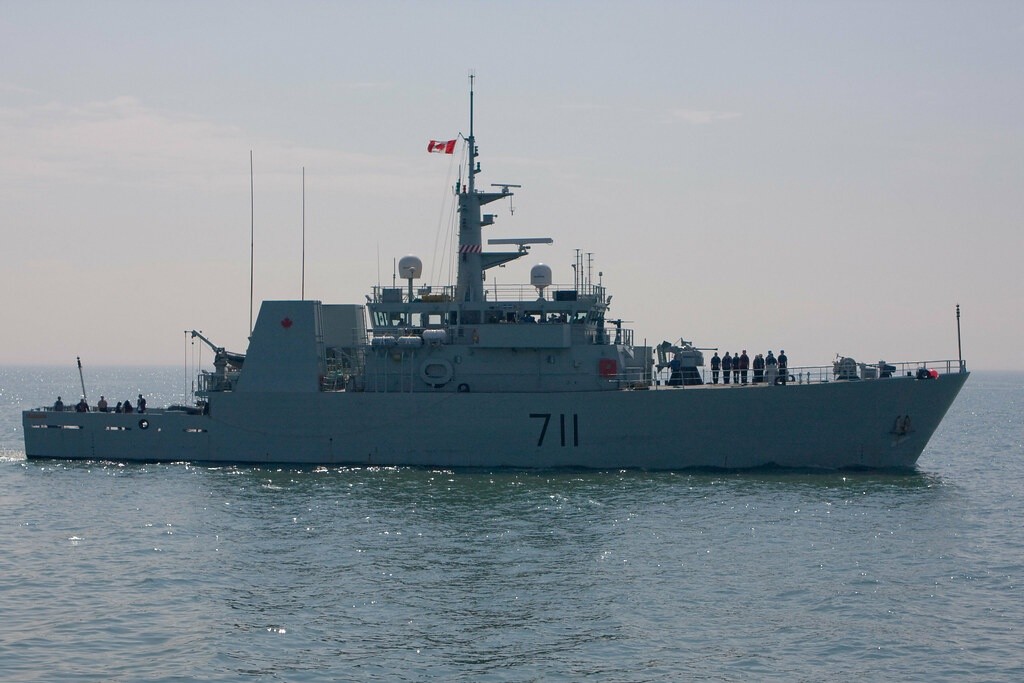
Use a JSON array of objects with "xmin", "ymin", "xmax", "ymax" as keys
[{"xmin": 428, "ymin": 140, "xmax": 456, "ymax": 155}]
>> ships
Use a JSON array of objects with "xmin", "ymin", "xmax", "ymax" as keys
[{"xmin": 22, "ymin": 73, "xmax": 973, "ymax": 472}]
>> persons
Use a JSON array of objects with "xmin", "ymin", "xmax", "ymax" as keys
[
  {"xmin": 777, "ymin": 350, "xmax": 788, "ymax": 382},
  {"xmin": 115, "ymin": 399, "xmax": 134, "ymax": 431},
  {"xmin": 74, "ymin": 399, "xmax": 90, "ymax": 429},
  {"xmin": 53, "ymin": 396, "xmax": 63, "ymax": 412},
  {"xmin": 711, "ymin": 352, "xmax": 721, "ymax": 384},
  {"xmin": 722, "ymin": 349, "xmax": 750, "ymax": 385},
  {"xmin": 97, "ymin": 396, "xmax": 108, "ymax": 412},
  {"xmin": 397, "ymin": 311, "xmax": 557, "ymax": 326},
  {"xmin": 136, "ymin": 394, "xmax": 146, "ymax": 413},
  {"xmin": 752, "ymin": 351, "xmax": 777, "ymax": 385}
]
